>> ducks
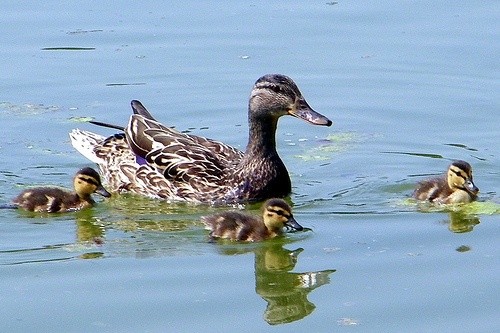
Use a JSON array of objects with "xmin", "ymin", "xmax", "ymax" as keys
[
  {"xmin": 7, "ymin": 166, "xmax": 112, "ymax": 213},
  {"xmin": 70, "ymin": 72, "xmax": 332, "ymax": 204},
  {"xmin": 200, "ymin": 198, "xmax": 305, "ymax": 243},
  {"xmin": 406, "ymin": 160, "xmax": 480, "ymax": 207}
]
